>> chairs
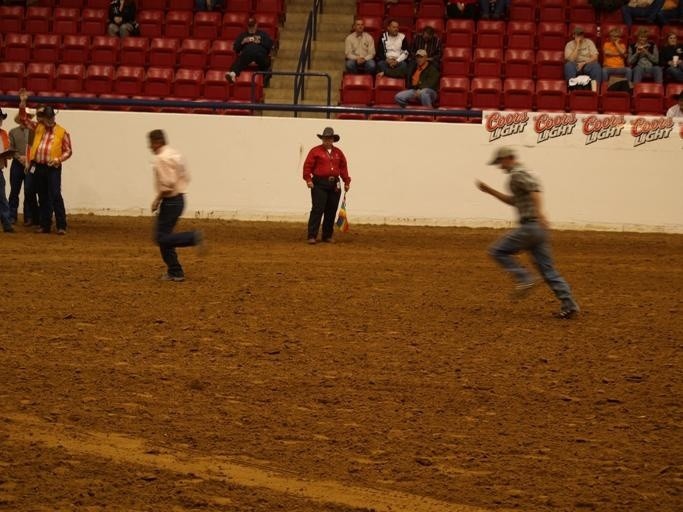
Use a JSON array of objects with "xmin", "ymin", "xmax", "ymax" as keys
[
  {"xmin": 335, "ymin": 1, "xmax": 683, "ymax": 122},
  {"xmin": 0, "ymin": 0, "xmax": 286, "ymax": 116}
]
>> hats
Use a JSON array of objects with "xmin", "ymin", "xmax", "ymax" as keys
[
  {"xmin": 488, "ymin": 147, "xmax": 513, "ymax": 164},
  {"xmin": 415, "ymin": 49, "xmax": 426, "ymax": 56},
  {"xmin": 317, "ymin": 128, "xmax": 339, "ymax": 141},
  {"xmin": 248, "ymin": 18, "xmax": 256, "ymax": 26},
  {"xmin": 0, "ymin": 107, "xmax": 55, "ymax": 123},
  {"xmin": 574, "ymin": 28, "xmax": 584, "ymax": 33}
]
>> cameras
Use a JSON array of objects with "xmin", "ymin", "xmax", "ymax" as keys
[{"xmin": 249, "ymin": 37, "xmax": 254, "ymax": 42}]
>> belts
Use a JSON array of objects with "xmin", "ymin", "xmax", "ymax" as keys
[{"xmin": 320, "ymin": 176, "xmax": 336, "ymax": 181}]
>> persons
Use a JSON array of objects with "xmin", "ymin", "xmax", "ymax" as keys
[
  {"xmin": 563, "ymin": 0, "xmax": 683, "ymax": 91},
  {"xmin": 447, "ymin": 0, "xmax": 507, "ymax": 20},
  {"xmin": 225, "ymin": 18, "xmax": 272, "ymax": 83},
  {"xmin": 375, "ymin": 20, "xmax": 416, "ymax": 79},
  {"xmin": 147, "ymin": 129, "xmax": 207, "ymax": 282},
  {"xmin": 394, "ymin": 49, "xmax": 440, "ymax": 107},
  {"xmin": 0, "ymin": 88, "xmax": 72, "ymax": 235},
  {"xmin": 412, "ymin": 27, "xmax": 443, "ymax": 70},
  {"xmin": 192, "ymin": 0, "xmax": 225, "ymax": 14},
  {"xmin": 107, "ymin": 0, "xmax": 136, "ymax": 37},
  {"xmin": 476, "ymin": 145, "xmax": 580, "ymax": 318},
  {"xmin": 302, "ymin": 127, "xmax": 351, "ymax": 244},
  {"xmin": 344, "ymin": 17, "xmax": 377, "ymax": 74}
]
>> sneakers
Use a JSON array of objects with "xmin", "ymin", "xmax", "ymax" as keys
[
  {"xmin": 161, "ymin": 273, "xmax": 184, "ymax": 281},
  {"xmin": 556, "ymin": 311, "xmax": 574, "ymax": 317},
  {"xmin": 225, "ymin": 74, "xmax": 235, "ymax": 83}
]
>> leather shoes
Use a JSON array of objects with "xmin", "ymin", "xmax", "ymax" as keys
[
  {"xmin": 24, "ymin": 219, "xmax": 66, "ymax": 234},
  {"xmin": 4, "ymin": 217, "xmax": 15, "ymax": 232},
  {"xmin": 309, "ymin": 239, "xmax": 316, "ymax": 243},
  {"xmin": 326, "ymin": 239, "xmax": 335, "ymax": 243}
]
